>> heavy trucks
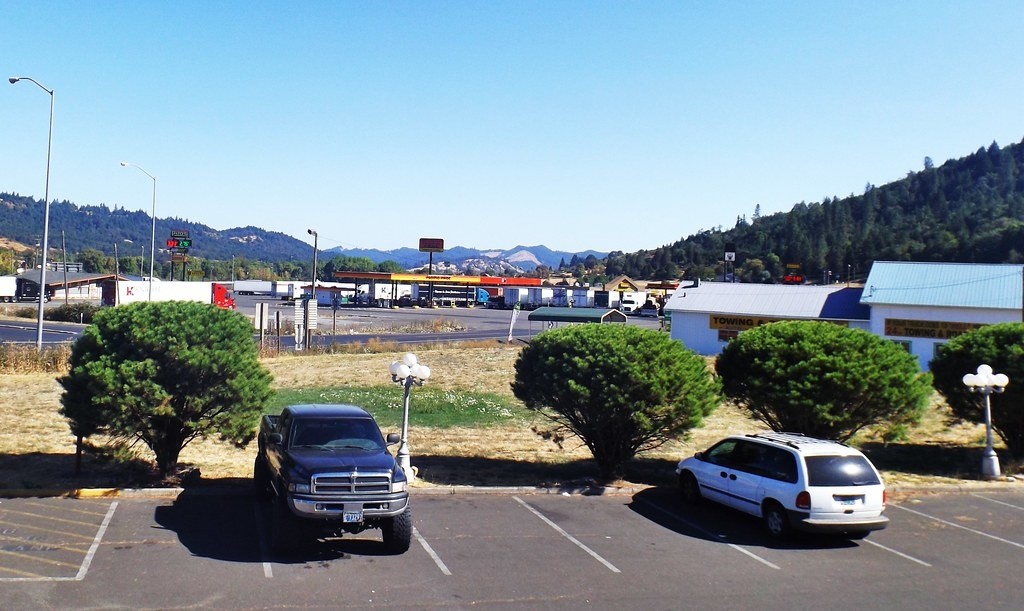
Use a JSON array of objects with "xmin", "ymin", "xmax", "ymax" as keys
[
  {"xmin": 232, "ymin": 279, "xmax": 672, "ymax": 319},
  {"xmin": 0, "ymin": 276, "xmax": 54, "ymax": 303}
]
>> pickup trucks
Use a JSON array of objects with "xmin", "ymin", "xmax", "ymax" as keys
[{"xmin": 253, "ymin": 401, "xmax": 414, "ymax": 554}]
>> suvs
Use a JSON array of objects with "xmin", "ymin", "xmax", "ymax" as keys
[{"xmin": 674, "ymin": 431, "xmax": 890, "ymax": 542}]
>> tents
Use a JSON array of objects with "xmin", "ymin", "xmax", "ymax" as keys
[{"xmin": 528, "ymin": 307, "xmax": 627, "ymax": 346}]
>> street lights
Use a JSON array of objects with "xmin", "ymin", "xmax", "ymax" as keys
[
  {"xmin": 8, "ymin": 77, "xmax": 56, "ymax": 353},
  {"xmin": 308, "ymin": 228, "xmax": 318, "ymax": 349},
  {"xmin": 962, "ymin": 364, "xmax": 1010, "ymax": 474},
  {"xmin": 120, "ymin": 161, "xmax": 157, "ymax": 302},
  {"xmin": 388, "ymin": 353, "xmax": 431, "ymax": 482}
]
